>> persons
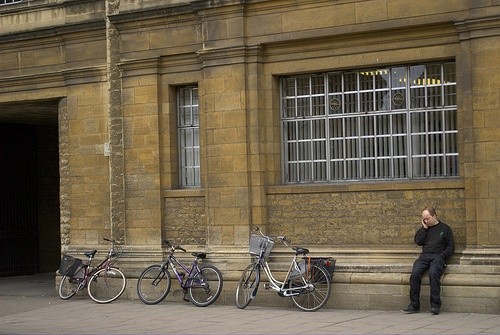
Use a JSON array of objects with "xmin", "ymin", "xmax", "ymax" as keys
[{"xmin": 404, "ymin": 207, "xmax": 455, "ymax": 314}]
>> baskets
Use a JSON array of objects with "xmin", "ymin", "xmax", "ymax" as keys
[
  {"xmin": 59, "ymin": 254, "xmax": 81, "ymax": 277},
  {"xmin": 249, "ymin": 234, "xmax": 274, "ymax": 259},
  {"xmin": 310, "ymin": 259, "xmax": 337, "ymax": 284}
]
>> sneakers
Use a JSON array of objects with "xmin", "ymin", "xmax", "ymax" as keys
[
  {"xmin": 401, "ymin": 305, "xmax": 420, "ymax": 314},
  {"xmin": 431, "ymin": 308, "xmax": 440, "ymax": 315}
]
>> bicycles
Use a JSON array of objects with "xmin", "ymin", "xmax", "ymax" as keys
[
  {"xmin": 59, "ymin": 236, "xmax": 127, "ymax": 304},
  {"xmin": 235, "ymin": 226, "xmax": 333, "ymax": 312},
  {"xmin": 137, "ymin": 239, "xmax": 223, "ymax": 307}
]
